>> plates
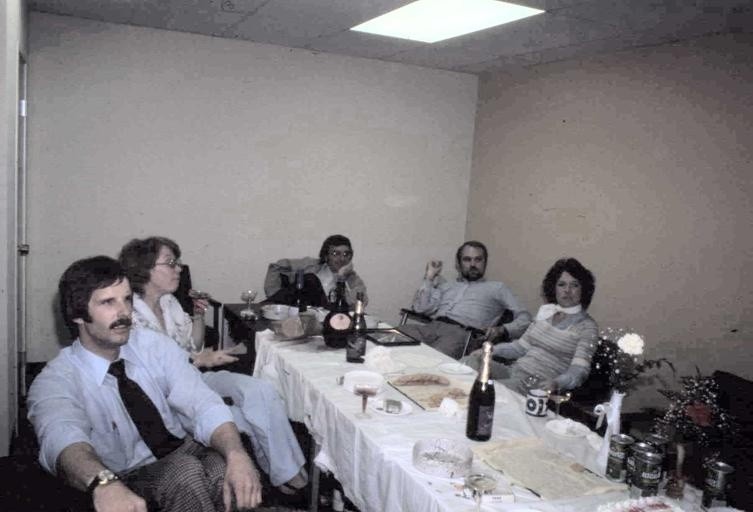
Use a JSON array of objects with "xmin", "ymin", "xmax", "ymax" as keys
[
  {"xmin": 366, "ymin": 358, "xmax": 407, "ymax": 374},
  {"xmin": 372, "ymin": 398, "xmax": 413, "ymax": 416},
  {"xmin": 341, "ymin": 370, "xmax": 387, "ymax": 394},
  {"xmin": 439, "ymin": 363, "xmax": 472, "ymax": 374},
  {"xmin": 544, "ymin": 419, "xmax": 590, "ymax": 437}
]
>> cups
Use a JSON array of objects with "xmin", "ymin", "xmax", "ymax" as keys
[{"xmin": 525, "ymin": 389, "xmax": 551, "ymax": 416}]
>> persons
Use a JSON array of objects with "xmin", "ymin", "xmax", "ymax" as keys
[
  {"xmin": 117, "ymin": 236, "xmax": 329, "ymax": 503},
  {"xmin": 262, "ymin": 235, "xmax": 369, "ymax": 315},
  {"xmin": 457, "ymin": 256, "xmax": 599, "ymax": 402},
  {"xmin": 26, "ymin": 256, "xmax": 266, "ymax": 512},
  {"xmin": 395, "ymin": 241, "xmax": 532, "ymax": 362}
]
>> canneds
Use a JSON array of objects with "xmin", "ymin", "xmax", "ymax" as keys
[
  {"xmin": 700, "ymin": 461, "xmax": 734, "ymax": 512},
  {"xmin": 604, "ymin": 433, "xmax": 634, "ymax": 483},
  {"xmin": 625, "ymin": 443, "xmax": 656, "ymax": 489},
  {"xmin": 630, "ymin": 451, "xmax": 661, "ymax": 498},
  {"xmin": 644, "ymin": 433, "xmax": 669, "ymax": 483}
]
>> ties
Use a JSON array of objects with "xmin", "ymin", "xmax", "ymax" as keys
[{"xmin": 107, "ymin": 359, "xmax": 186, "ymax": 460}]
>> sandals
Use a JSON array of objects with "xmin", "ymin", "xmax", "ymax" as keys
[{"xmin": 279, "ymin": 480, "xmax": 333, "ymax": 510}]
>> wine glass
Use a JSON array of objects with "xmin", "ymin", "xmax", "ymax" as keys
[
  {"xmin": 546, "ymin": 378, "xmax": 572, "ymax": 422},
  {"xmin": 353, "ymin": 382, "xmax": 378, "ymax": 420},
  {"xmin": 240, "ymin": 289, "xmax": 258, "ymax": 315},
  {"xmin": 187, "ymin": 288, "xmax": 211, "ymax": 320}
]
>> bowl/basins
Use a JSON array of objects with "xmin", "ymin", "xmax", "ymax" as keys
[
  {"xmin": 364, "ymin": 318, "xmax": 379, "ymax": 330},
  {"xmin": 261, "ymin": 304, "xmax": 289, "ymax": 320}
]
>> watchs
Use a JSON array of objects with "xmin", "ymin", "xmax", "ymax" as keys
[{"xmin": 85, "ymin": 469, "xmax": 118, "ymax": 494}]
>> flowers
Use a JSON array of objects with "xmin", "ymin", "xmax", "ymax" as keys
[{"xmin": 586, "ymin": 324, "xmax": 741, "ymax": 500}]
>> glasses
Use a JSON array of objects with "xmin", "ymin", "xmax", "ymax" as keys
[
  {"xmin": 329, "ymin": 250, "xmax": 352, "ymax": 257},
  {"xmin": 155, "ymin": 258, "xmax": 182, "ymax": 268}
]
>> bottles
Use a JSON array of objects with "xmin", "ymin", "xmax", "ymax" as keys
[
  {"xmin": 322, "ymin": 280, "xmax": 353, "ymax": 349},
  {"xmin": 290, "ymin": 269, "xmax": 307, "ymax": 313},
  {"xmin": 466, "ymin": 340, "xmax": 495, "ymax": 442},
  {"xmin": 346, "ymin": 292, "xmax": 367, "ymax": 364}
]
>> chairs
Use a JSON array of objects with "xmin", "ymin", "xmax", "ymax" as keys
[
  {"xmin": 397, "ymin": 308, "xmax": 512, "ymax": 365},
  {"xmin": 545, "ymin": 337, "xmax": 623, "ymax": 437},
  {"xmin": 172, "ymin": 264, "xmax": 221, "ymax": 350},
  {"xmin": 664, "ymin": 368, "xmax": 753, "ymax": 512},
  {"xmin": 264, "ymin": 257, "xmax": 321, "ymax": 303}
]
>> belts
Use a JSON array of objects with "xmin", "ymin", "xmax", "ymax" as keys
[{"xmin": 436, "ymin": 316, "xmax": 485, "ymax": 335}]
